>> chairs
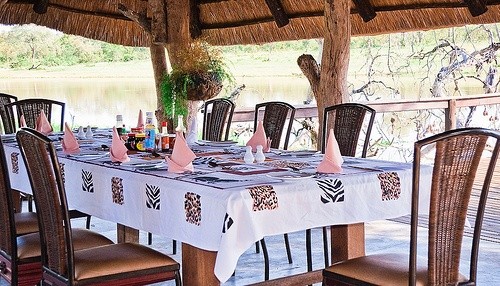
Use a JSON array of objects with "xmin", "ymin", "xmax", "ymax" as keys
[{"xmin": 0, "ymin": 93, "xmax": 500, "ymax": 286}]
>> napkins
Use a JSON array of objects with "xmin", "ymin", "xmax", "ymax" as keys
[
  {"xmin": 165, "ymin": 129, "xmax": 197, "ymax": 173},
  {"xmin": 60, "ymin": 122, "xmax": 81, "ymax": 156},
  {"xmin": 33, "ymin": 108, "xmax": 53, "ymax": 137},
  {"xmin": 136, "ymin": 109, "xmax": 145, "ymax": 129},
  {"xmin": 246, "ymin": 120, "xmax": 272, "ymax": 153},
  {"xmin": 109, "ymin": 125, "xmax": 131, "ymax": 163},
  {"xmin": 20, "ymin": 113, "xmax": 27, "ymax": 128},
  {"xmin": 315, "ymin": 128, "xmax": 344, "ymax": 174}
]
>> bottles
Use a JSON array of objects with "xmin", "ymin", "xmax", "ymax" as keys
[
  {"xmin": 244, "ymin": 145, "xmax": 254, "ymax": 163},
  {"xmin": 86, "ymin": 126, "xmax": 93, "ymax": 138},
  {"xmin": 115, "ymin": 111, "xmax": 155, "ymax": 150},
  {"xmin": 160, "ymin": 122, "xmax": 169, "ymax": 152},
  {"xmin": 78, "ymin": 126, "xmax": 85, "ymax": 140},
  {"xmin": 175, "ymin": 115, "xmax": 186, "ymax": 139},
  {"xmin": 255, "ymin": 145, "xmax": 265, "ymax": 162}
]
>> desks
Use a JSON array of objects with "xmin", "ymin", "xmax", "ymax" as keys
[{"xmin": 0, "ymin": 125, "xmax": 472, "ymax": 286}]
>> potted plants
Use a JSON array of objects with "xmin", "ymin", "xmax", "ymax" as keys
[{"xmin": 159, "ymin": 35, "xmax": 237, "ymax": 127}]
